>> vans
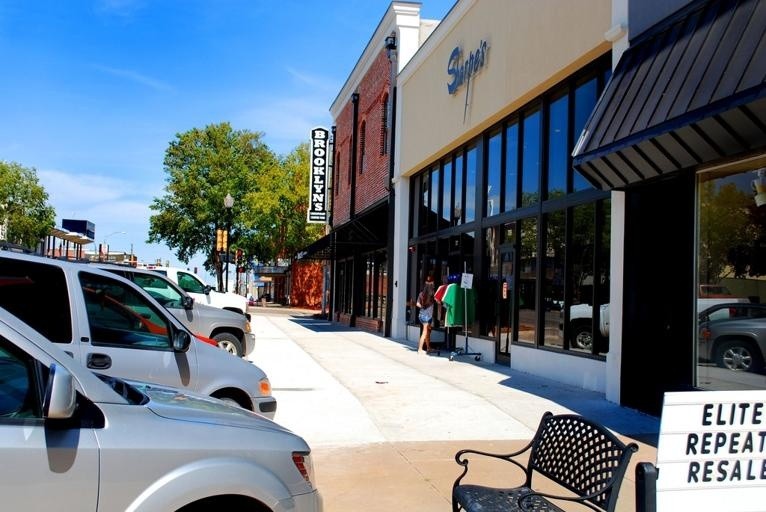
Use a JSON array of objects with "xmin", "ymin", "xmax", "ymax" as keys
[{"xmin": 0, "ymin": 305, "xmax": 327, "ymax": 512}]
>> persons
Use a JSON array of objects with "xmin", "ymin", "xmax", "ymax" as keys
[
  {"xmin": 415, "ymin": 280, "xmax": 442, "ymax": 356},
  {"xmin": 247, "ymin": 288, "xmax": 270, "ymax": 307}
]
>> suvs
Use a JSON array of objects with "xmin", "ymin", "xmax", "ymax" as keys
[
  {"xmin": 27, "ymin": 254, "xmax": 255, "ymax": 360},
  {"xmin": 1, "ymin": 249, "xmax": 278, "ymax": 418}
]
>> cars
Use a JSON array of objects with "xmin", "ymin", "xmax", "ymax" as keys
[{"xmin": 548, "ymin": 282, "xmax": 765, "ymax": 374}]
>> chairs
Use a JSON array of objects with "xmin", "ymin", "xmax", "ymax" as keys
[{"xmin": 452, "ymin": 411, "xmax": 639, "ymax": 510}]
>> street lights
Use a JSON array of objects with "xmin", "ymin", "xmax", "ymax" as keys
[
  {"xmin": 103, "ymin": 230, "xmax": 125, "ymax": 262},
  {"xmin": 222, "ymin": 192, "xmax": 234, "ymax": 292}
]
